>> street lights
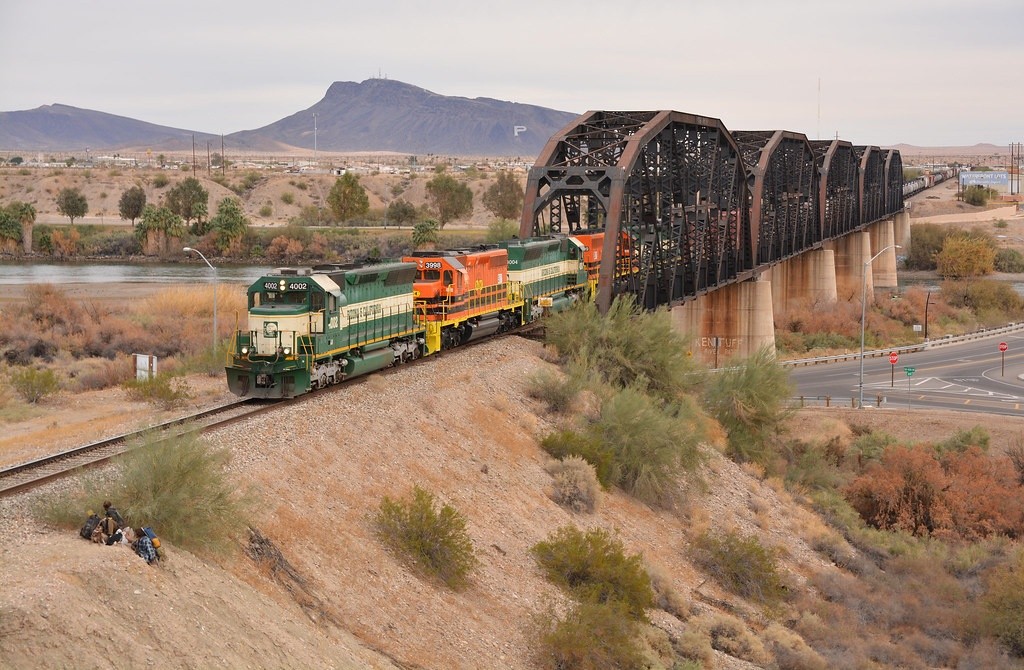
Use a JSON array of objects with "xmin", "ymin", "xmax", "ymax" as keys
[
  {"xmin": 183, "ymin": 247, "xmax": 217, "ymax": 377},
  {"xmin": 858, "ymin": 245, "xmax": 903, "ymax": 410}
]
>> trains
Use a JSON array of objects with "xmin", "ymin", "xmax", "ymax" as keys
[{"xmin": 224, "ymin": 168, "xmax": 958, "ymax": 399}]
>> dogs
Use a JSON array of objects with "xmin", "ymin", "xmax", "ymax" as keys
[
  {"xmin": 105, "ymin": 528, "xmax": 123, "ymax": 547},
  {"xmin": 89, "ymin": 525, "xmax": 108, "ymax": 546}
]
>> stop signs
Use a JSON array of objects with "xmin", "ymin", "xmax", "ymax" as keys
[
  {"xmin": 888, "ymin": 352, "xmax": 898, "ymax": 364},
  {"xmin": 999, "ymin": 343, "xmax": 1008, "ymax": 352}
]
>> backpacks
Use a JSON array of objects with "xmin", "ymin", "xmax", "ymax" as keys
[
  {"xmin": 141, "ymin": 527, "xmax": 161, "ymax": 548},
  {"xmin": 80, "ymin": 514, "xmax": 99, "ymax": 539},
  {"xmin": 91, "ymin": 525, "xmax": 104, "ymax": 543}
]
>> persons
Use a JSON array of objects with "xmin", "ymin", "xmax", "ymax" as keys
[
  {"xmin": 80, "ymin": 501, "xmax": 124, "ymax": 545},
  {"xmin": 132, "ymin": 528, "xmax": 155, "ymax": 564}
]
